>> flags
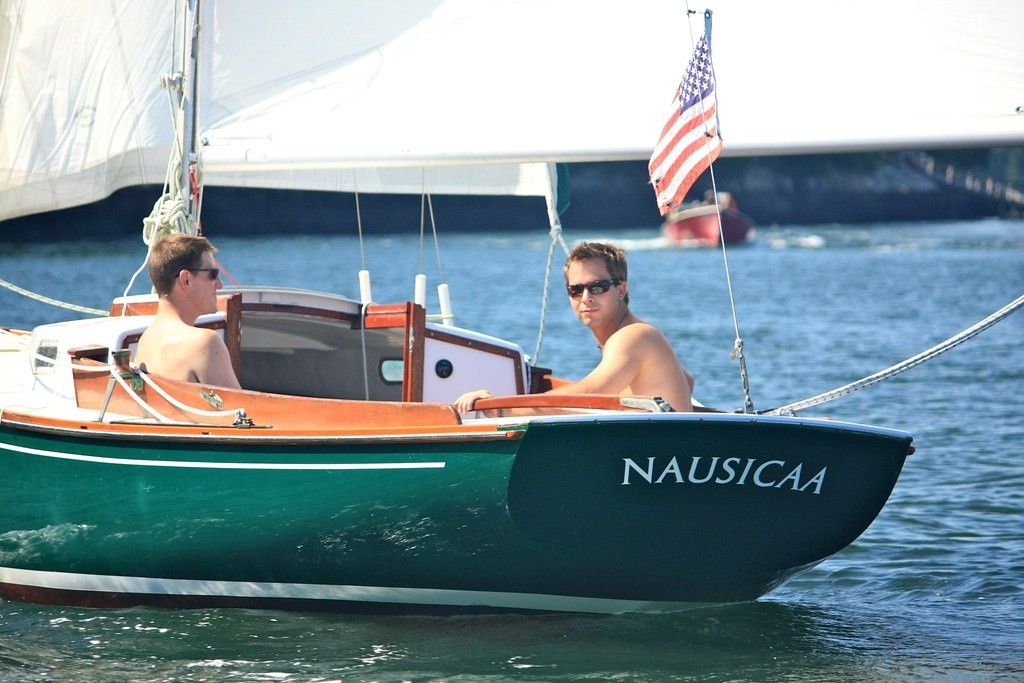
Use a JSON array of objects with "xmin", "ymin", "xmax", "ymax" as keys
[{"xmin": 649, "ymin": 9, "xmax": 722, "ymax": 215}]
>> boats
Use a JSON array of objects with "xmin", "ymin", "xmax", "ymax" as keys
[{"xmin": 667, "ymin": 205, "xmax": 754, "ymax": 248}]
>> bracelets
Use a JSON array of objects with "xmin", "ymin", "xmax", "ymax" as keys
[{"xmin": 497, "ymin": 409, "xmax": 504, "ymax": 417}]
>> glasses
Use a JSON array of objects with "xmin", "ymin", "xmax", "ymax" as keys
[
  {"xmin": 175, "ymin": 268, "xmax": 219, "ymax": 282},
  {"xmin": 567, "ymin": 279, "xmax": 617, "ymax": 298}
]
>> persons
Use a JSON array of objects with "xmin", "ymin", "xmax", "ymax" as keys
[
  {"xmin": 454, "ymin": 242, "xmax": 695, "ymax": 418},
  {"xmin": 135, "ymin": 234, "xmax": 242, "ymax": 389}
]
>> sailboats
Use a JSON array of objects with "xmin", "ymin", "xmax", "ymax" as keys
[{"xmin": 1, "ymin": 1, "xmax": 915, "ymax": 621}]
